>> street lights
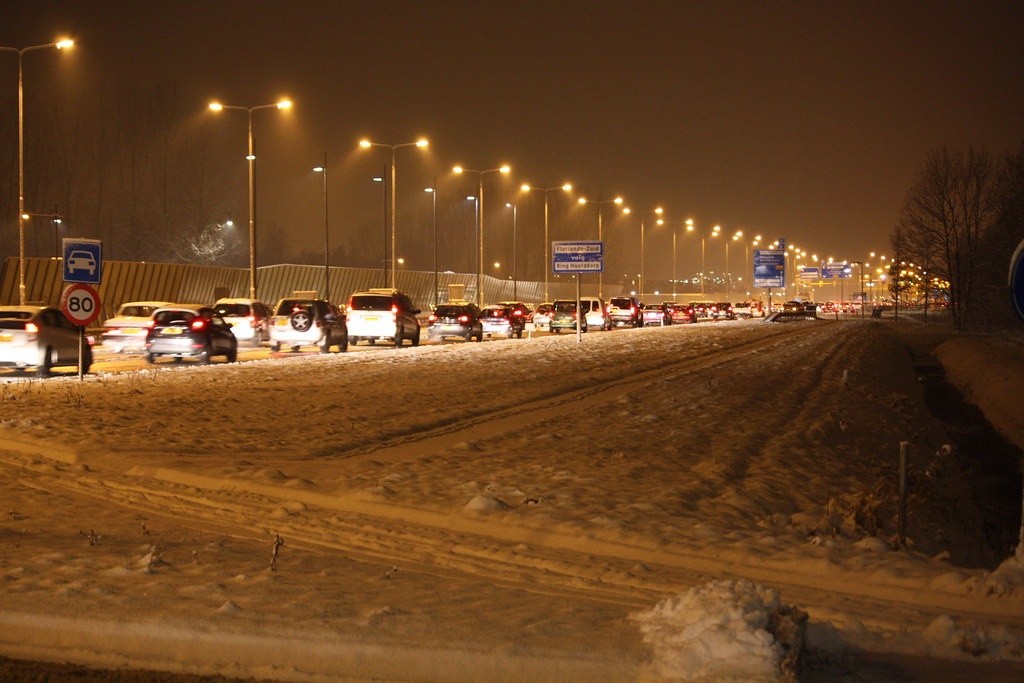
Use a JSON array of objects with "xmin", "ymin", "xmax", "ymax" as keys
[
  {"xmin": 206, "ymin": 96, "xmax": 292, "ymax": 300},
  {"xmin": 1, "ymin": 38, "xmax": 75, "ymax": 304},
  {"xmin": 452, "ymin": 164, "xmax": 849, "ymax": 298},
  {"xmin": 361, "ymin": 138, "xmax": 440, "ymax": 302},
  {"xmin": 852, "ymin": 261, "xmax": 865, "ymax": 317},
  {"xmin": 313, "ymin": 165, "xmax": 330, "ymax": 302}
]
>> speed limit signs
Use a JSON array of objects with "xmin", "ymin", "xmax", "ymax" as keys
[{"xmin": 62, "ymin": 284, "xmax": 101, "ymax": 325}]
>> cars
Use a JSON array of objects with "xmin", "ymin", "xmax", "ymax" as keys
[
  {"xmin": 0, "ymin": 306, "xmax": 95, "ymax": 377},
  {"xmin": 550, "ymin": 301, "xmax": 588, "ymax": 334},
  {"xmin": 146, "ymin": 304, "xmax": 240, "ymax": 366},
  {"xmin": 642, "ymin": 300, "xmax": 892, "ymax": 327},
  {"xmin": 534, "ymin": 304, "xmax": 554, "ymax": 327},
  {"xmin": 480, "ymin": 301, "xmax": 534, "ymax": 339},
  {"xmin": 213, "ymin": 297, "xmax": 273, "ymax": 345},
  {"xmin": 428, "ymin": 305, "xmax": 483, "ymax": 341},
  {"xmin": 101, "ymin": 300, "xmax": 177, "ymax": 354}
]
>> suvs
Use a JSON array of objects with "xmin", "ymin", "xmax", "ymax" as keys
[
  {"xmin": 267, "ymin": 298, "xmax": 349, "ymax": 354},
  {"xmin": 606, "ymin": 297, "xmax": 645, "ymax": 328}
]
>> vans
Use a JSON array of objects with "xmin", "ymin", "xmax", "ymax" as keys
[
  {"xmin": 344, "ymin": 287, "xmax": 423, "ymax": 347},
  {"xmin": 578, "ymin": 296, "xmax": 612, "ymax": 332}
]
